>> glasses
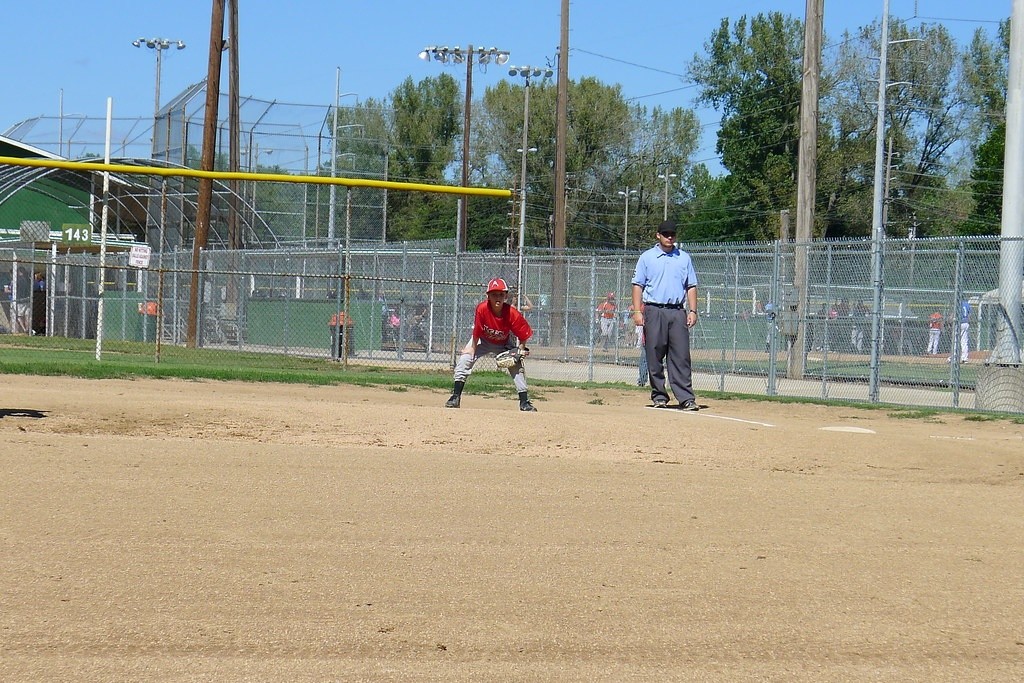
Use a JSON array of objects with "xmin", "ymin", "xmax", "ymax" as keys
[{"xmin": 661, "ymin": 232, "xmax": 676, "ymax": 237}]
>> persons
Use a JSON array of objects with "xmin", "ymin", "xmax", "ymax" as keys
[
  {"xmin": 622, "ymin": 298, "xmax": 648, "ymax": 387},
  {"xmin": 4, "ymin": 267, "xmax": 45, "ymax": 336},
  {"xmin": 945, "ymin": 294, "xmax": 973, "ymax": 364},
  {"xmin": 444, "ymin": 276, "xmax": 550, "ymax": 412},
  {"xmin": 764, "ymin": 296, "xmax": 870, "ymax": 354},
  {"xmin": 631, "ymin": 220, "xmax": 702, "ymax": 411},
  {"xmin": 595, "ymin": 292, "xmax": 618, "ymax": 352},
  {"xmin": 926, "ymin": 306, "xmax": 944, "ymax": 356},
  {"xmin": 390, "ymin": 305, "xmax": 405, "ymax": 347}
]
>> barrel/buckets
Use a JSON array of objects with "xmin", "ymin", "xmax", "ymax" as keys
[
  {"xmin": 135, "ymin": 311, "xmax": 161, "ymax": 342},
  {"xmin": 329, "ymin": 322, "xmax": 356, "ymax": 358}
]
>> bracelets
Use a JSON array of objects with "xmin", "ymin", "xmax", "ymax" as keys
[{"xmin": 689, "ymin": 309, "xmax": 697, "ymax": 315}]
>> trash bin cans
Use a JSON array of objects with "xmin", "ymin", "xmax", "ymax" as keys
[
  {"xmin": 328, "ymin": 310, "xmax": 355, "ymax": 357},
  {"xmin": 137, "ymin": 302, "xmax": 163, "ymax": 343}
]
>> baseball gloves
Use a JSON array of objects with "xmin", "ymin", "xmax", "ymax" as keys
[{"xmin": 496, "ymin": 347, "xmax": 530, "ymax": 368}]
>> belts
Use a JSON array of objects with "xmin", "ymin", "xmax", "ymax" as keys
[{"xmin": 646, "ymin": 303, "xmax": 684, "ymax": 309}]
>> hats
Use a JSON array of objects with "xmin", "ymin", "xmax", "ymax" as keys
[
  {"xmin": 607, "ymin": 293, "xmax": 615, "ymax": 298},
  {"xmin": 658, "ymin": 221, "xmax": 678, "ymax": 234},
  {"xmin": 486, "ymin": 279, "xmax": 508, "ymax": 292}
]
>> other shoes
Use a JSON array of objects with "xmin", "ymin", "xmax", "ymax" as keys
[
  {"xmin": 446, "ymin": 394, "xmax": 460, "ymax": 407},
  {"xmin": 683, "ymin": 401, "xmax": 700, "ymax": 410},
  {"xmin": 520, "ymin": 400, "xmax": 537, "ymax": 412},
  {"xmin": 654, "ymin": 400, "xmax": 666, "ymax": 408}
]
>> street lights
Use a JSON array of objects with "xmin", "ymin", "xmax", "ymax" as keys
[
  {"xmin": 507, "ymin": 65, "xmax": 553, "ymax": 346},
  {"xmin": 241, "ymin": 150, "xmax": 273, "ymax": 244},
  {"xmin": 657, "ymin": 173, "xmax": 677, "ymax": 221},
  {"xmin": 617, "ymin": 191, "xmax": 636, "ymax": 254},
  {"xmin": 418, "ymin": 44, "xmax": 509, "ymax": 344},
  {"xmin": 132, "ymin": 36, "xmax": 187, "ymax": 187}
]
